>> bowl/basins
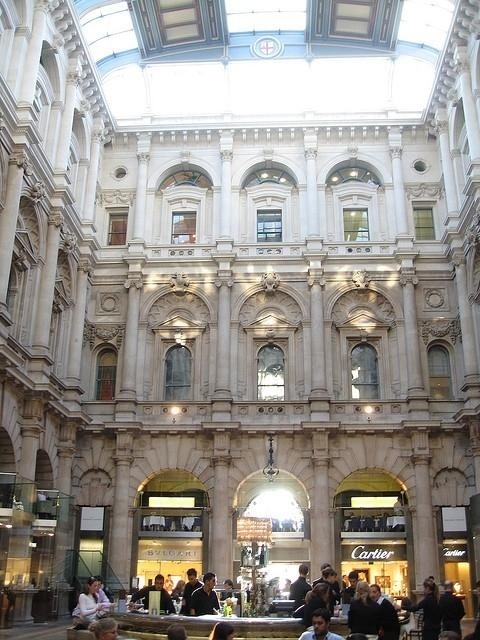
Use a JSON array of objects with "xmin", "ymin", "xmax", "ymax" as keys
[{"xmin": 127, "ymin": 603, "xmax": 148, "ymax": 614}]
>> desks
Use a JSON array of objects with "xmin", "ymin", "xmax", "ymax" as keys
[
  {"xmin": 34, "ymin": 501, "xmax": 54, "ymax": 520},
  {"xmin": 270, "ymin": 517, "xmax": 297, "ymax": 532},
  {"xmin": 139, "ymin": 514, "xmax": 202, "ymax": 533},
  {"xmin": 341, "ymin": 512, "xmax": 406, "ymax": 533}
]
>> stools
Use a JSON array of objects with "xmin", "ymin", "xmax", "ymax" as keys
[{"xmin": 64, "ymin": 627, "xmax": 98, "ymax": 640}]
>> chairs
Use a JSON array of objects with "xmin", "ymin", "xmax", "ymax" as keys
[
  {"xmin": 52, "ymin": 502, "xmax": 57, "ymax": 519},
  {"xmin": 408, "ymin": 611, "xmax": 425, "ymax": 640}
]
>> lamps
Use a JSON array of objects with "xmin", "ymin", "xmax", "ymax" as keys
[
  {"xmin": 260, "ymin": 434, "xmax": 283, "ymax": 482},
  {"xmin": 233, "ymin": 515, "xmax": 275, "ymax": 587}
]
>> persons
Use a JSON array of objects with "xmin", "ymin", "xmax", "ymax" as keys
[
  {"xmin": 128, "ymin": 569, "xmax": 233, "ymax": 617},
  {"xmin": 167, "ymin": 624, "xmax": 187, "ymax": 639},
  {"xmin": 72, "ymin": 575, "xmax": 118, "ymax": 639},
  {"xmin": 208, "ymin": 622, "xmax": 234, "ymax": 639},
  {"xmin": 282, "ymin": 563, "xmax": 467, "ymax": 640}
]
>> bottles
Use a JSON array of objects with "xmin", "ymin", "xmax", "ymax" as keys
[
  {"xmin": 224, "ymin": 605, "xmax": 226, "ymax": 617},
  {"xmin": 243, "ymin": 610, "xmax": 248, "ymax": 617},
  {"xmin": 118, "ymin": 598, "xmax": 126, "ymax": 614},
  {"xmin": 274, "ymin": 589, "xmax": 281, "ymax": 600},
  {"xmin": 227, "ymin": 605, "xmax": 232, "ymax": 617},
  {"xmin": 109, "ymin": 603, "xmax": 117, "ymax": 613},
  {"xmin": 127, "ymin": 593, "xmax": 132, "ymax": 602},
  {"xmin": 339, "ymin": 608, "xmax": 343, "ymax": 618}
]
